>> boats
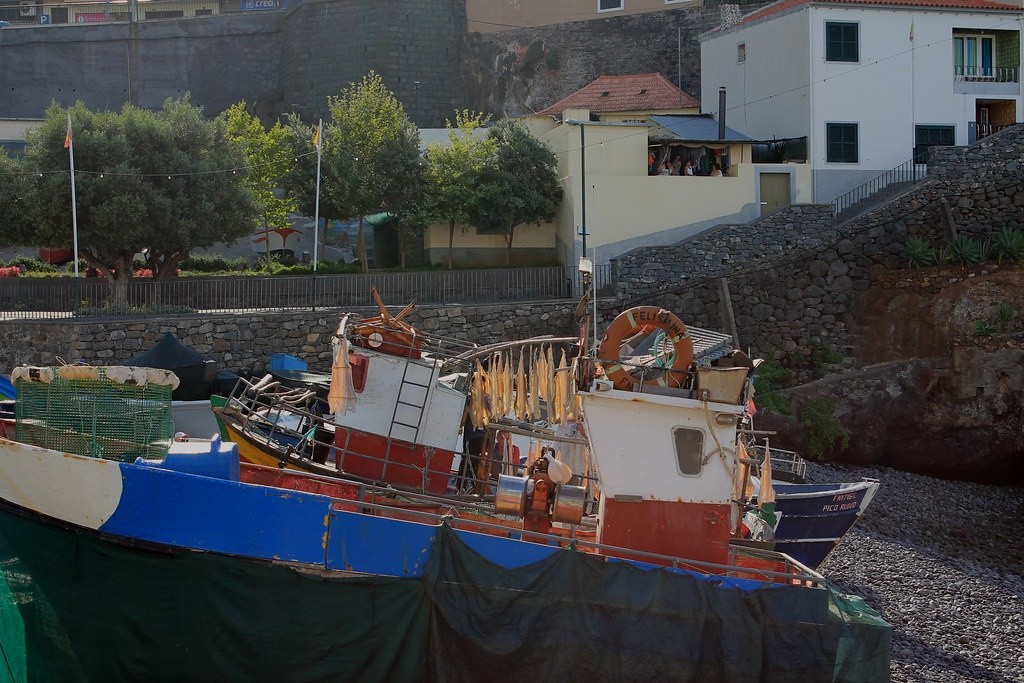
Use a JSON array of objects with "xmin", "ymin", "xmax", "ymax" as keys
[{"xmin": 211, "ymin": 310, "xmax": 879, "ymax": 573}]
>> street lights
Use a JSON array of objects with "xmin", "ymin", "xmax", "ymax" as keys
[{"xmin": 564, "ymin": 118, "xmax": 589, "ymax": 298}]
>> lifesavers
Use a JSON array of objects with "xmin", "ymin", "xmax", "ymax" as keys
[{"xmin": 599, "ymin": 305, "xmax": 694, "ymax": 392}]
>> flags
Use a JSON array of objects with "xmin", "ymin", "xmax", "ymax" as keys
[
  {"xmin": 64, "ymin": 130, "xmax": 72, "ymax": 148},
  {"xmin": 910, "ymin": 27, "xmax": 913, "ymax": 41},
  {"xmin": 313, "ymin": 128, "xmax": 320, "ymax": 145}
]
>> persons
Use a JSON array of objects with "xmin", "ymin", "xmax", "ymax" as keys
[
  {"xmin": 490, "ymin": 430, "xmax": 521, "ymax": 480},
  {"xmin": 456, "ymin": 376, "xmax": 492, "ymax": 487},
  {"xmin": 659, "ymin": 156, "xmax": 723, "ymax": 177}
]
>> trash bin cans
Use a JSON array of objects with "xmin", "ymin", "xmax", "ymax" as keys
[{"xmin": 302, "ymin": 251, "xmax": 311, "ymax": 262}]
